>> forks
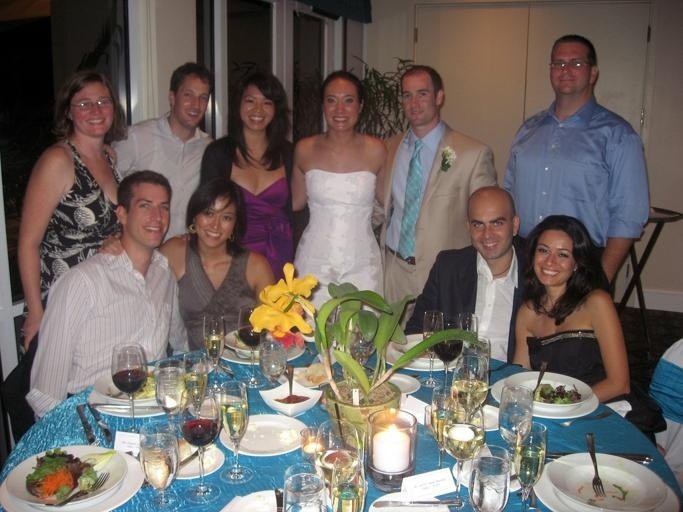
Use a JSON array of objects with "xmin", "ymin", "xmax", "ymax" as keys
[
  {"xmin": 288, "ymin": 365, "xmax": 295, "ymax": 395},
  {"xmin": 532, "ymin": 362, "xmax": 546, "ymax": 397},
  {"xmin": 585, "ymin": 431, "xmax": 606, "ymax": 497},
  {"xmin": 551, "ymin": 411, "xmax": 611, "ymax": 428}
]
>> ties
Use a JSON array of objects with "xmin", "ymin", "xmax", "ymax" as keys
[{"xmin": 399, "ymin": 139, "xmax": 424, "ymax": 259}]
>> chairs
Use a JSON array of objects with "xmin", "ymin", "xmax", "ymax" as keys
[{"xmin": 646, "ymin": 337, "xmax": 683, "ymax": 477}]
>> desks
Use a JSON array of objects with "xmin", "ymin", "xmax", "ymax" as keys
[{"xmin": 617, "ymin": 203, "xmax": 682, "ymax": 315}]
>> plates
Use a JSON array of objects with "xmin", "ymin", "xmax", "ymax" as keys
[
  {"xmin": 0, "ymin": 445, "xmax": 144, "ymax": 512},
  {"xmin": 276, "ymin": 367, "xmax": 329, "ymax": 389},
  {"xmin": 222, "ymin": 414, "xmax": 309, "ymax": 459},
  {"xmin": 490, "ymin": 371, "xmax": 599, "ymax": 419},
  {"xmin": 533, "ymin": 453, "xmax": 681, "ymax": 512},
  {"xmin": 385, "ymin": 333, "xmax": 470, "ymax": 369},
  {"xmin": 369, "ymin": 492, "xmax": 451, "ymax": 511},
  {"xmin": 368, "ymin": 371, "xmax": 420, "ymax": 394},
  {"xmin": 172, "ymin": 437, "xmax": 223, "ymax": 481},
  {"xmin": 88, "ymin": 365, "xmax": 185, "ymax": 417}
]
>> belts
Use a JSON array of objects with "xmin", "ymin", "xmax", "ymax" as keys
[{"xmin": 388, "ymin": 246, "xmax": 415, "ymax": 264}]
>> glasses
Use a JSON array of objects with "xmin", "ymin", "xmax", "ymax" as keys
[
  {"xmin": 549, "ymin": 61, "xmax": 589, "ymax": 69},
  {"xmin": 70, "ymin": 99, "xmax": 113, "ymax": 108}
]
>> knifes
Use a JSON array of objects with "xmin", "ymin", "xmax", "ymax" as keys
[{"xmin": 373, "ymin": 499, "xmax": 464, "ymax": 508}]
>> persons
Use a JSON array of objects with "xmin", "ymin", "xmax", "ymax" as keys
[
  {"xmin": 110, "ymin": 63, "xmax": 216, "ymax": 244},
  {"xmin": 515, "ymin": 215, "xmax": 632, "ymax": 419},
  {"xmin": 25, "ymin": 170, "xmax": 191, "ymax": 421},
  {"xmin": 201, "ymin": 68, "xmax": 303, "ymax": 277},
  {"xmin": 504, "ymin": 34, "xmax": 650, "ymax": 286},
  {"xmin": 382, "ymin": 66, "xmax": 498, "ymax": 313},
  {"xmin": 98, "ymin": 178, "xmax": 276, "ymax": 357},
  {"xmin": 290, "ymin": 70, "xmax": 388, "ymax": 315},
  {"xmin": 18, "ymin": 70, "xmax": 124, "ymax": 350},
  {"xmin": 404, "ymin": 187, "xmax": 539, "ymax": 365}
]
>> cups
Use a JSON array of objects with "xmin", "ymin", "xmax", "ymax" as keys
[
  {"xmin": 368, "ymin": 409, "xmax": 417, "ymax": 493},
  {"xmin": 282, "ymin": 419, "xmax": 368, "ymax": 511},
  {"xmin": 470, "ymin": 444, "xmax": 513, "ymax": 512}
]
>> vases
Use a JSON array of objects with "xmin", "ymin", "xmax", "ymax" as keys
[{"xmin": 324, "ymin": 380, "xmax": 404, "ymax": 446}]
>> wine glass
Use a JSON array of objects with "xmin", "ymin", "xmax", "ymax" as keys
[
  {"xmin": 514, "ymin": 419, "xmax": 547, "ymax": 512},
  {"xmin": 139, "ymin": 419, "xmax": 182, "ymax": 512},
  {"xmin": 153, "ymin": 312, "xmax": 285, "ymax": 434},
  {"xmin": 111, "ymin": 342, "xmax": 148, "ymax": 433},
  {"xmin": 180, "ymin": 387, "xmax": 223, "ymax": 505},
  {"xmin": 425, "ymin": 312, "xmax": 491, "ymax": 507},
  {"xmin": 221, "ymin": 380, "xmax": 254, "ymax": 483}
]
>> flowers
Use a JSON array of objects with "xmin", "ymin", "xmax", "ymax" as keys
[{"xmin": 248, "ymin": 260, "xmax": 482, "ymax": 395}]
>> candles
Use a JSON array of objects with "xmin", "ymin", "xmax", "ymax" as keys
[{"xmin": 371, "ymin": 423, "xmax": 410, "ymax": 472}]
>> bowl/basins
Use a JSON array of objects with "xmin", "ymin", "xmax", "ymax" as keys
[{"xmin": 258, "ymin": 381, "xmax": 323, "ymax": 418}]
